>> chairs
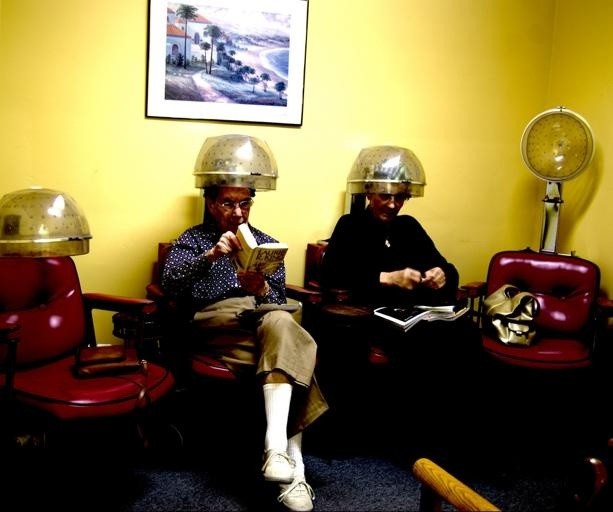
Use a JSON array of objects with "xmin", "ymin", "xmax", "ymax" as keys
[
  {"xmin": 466, "ymin": 245, "xmax": 613, "ymax": 374},
  {"xmin": 111, "ymin": 244, "xmax": 320, "ymax": 385},
  {"xmin": 302, "ymin": 239, "xmax": 464, "ymax": 366},
  {"xmin": 0, "ymin": 250, "xmax": 173, "ymax": 501}
]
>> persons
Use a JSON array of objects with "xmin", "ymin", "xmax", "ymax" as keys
[
  {"xmin": 159, "ymin": 186, "xmax": 330, "ymax": 512},
  {"xmin": 319, "ymin": 156, "xmax": 515, "ymax": 491}
]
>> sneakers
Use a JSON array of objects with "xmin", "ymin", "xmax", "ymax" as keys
[{"xmin": 262, "ymin": 450, "xmax": 315, "ymax": 512}]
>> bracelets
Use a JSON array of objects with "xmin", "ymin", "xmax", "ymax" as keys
[{"xmin": 257, "ymin": 280, "xmax": 269, "ymax": 296}]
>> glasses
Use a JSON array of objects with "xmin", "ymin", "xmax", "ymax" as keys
[{"xmin": 217, "ymin": 199, "xmax": 254, "ymax": 209}]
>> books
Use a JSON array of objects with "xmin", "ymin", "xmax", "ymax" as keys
[
  {"xmin": 373, "ymin": 302, "xmax": 470, "ymax": 333},
  {"xmin": 229, "ymin": 221, "xmax": 289, "ymax": 276}
]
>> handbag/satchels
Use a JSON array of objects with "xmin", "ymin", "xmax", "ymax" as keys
[{"xmin": 484, "ymin": 284, "xmax": 539, "ymax": 346}]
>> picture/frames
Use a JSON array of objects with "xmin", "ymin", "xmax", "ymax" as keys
[{"xmin": 142, "ymin": 0, "xmax": 310, "ymax": 129}]
